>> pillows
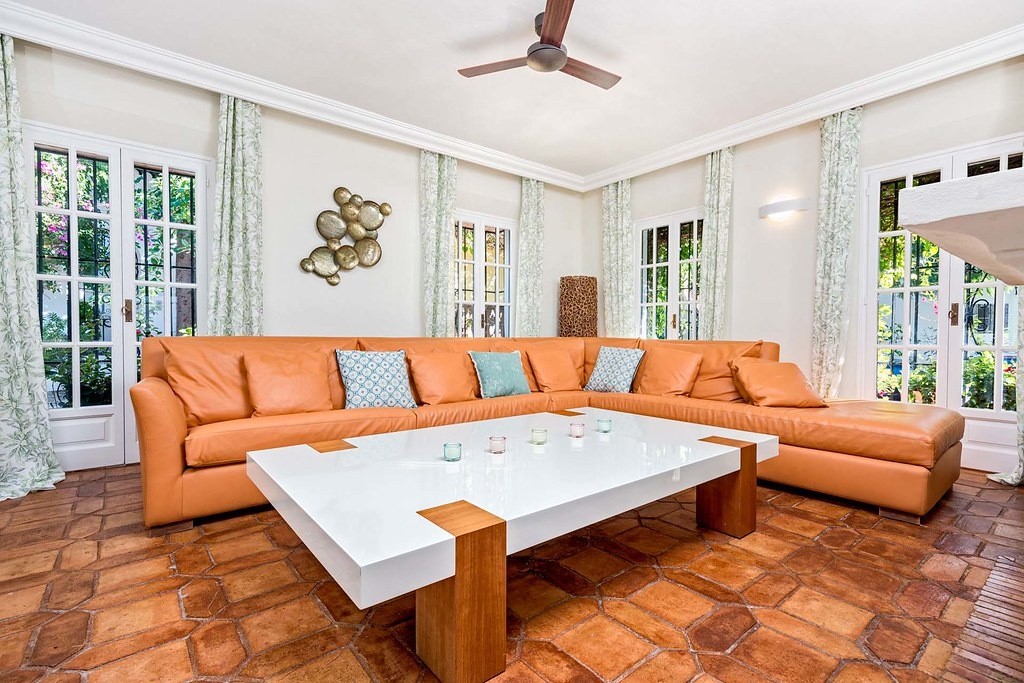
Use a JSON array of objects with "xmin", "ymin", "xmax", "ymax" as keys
[
  {"xmin": 407, "ymin": 354, "xmax": 475, "ymax": 406},
  {"xmin": 638, "ymin": 341, "xmax": 763, "ymax": 398},
  {"xmin": 636, "ymin": 347, "xmax": 701, "ymax": 397},
  {"xmin": 243, "ymin": 351, "xmax": 333, "ymax": 417},
  {"xmin": 527, "ymin": 349, "xmax": 582, "ymax": 392},
  {"xmin": 336, "ymin": 349, "xmax": 417, "ymax": 410},
  {"xmin": 160, "ymin": 337, "xmax": 357, "ymax": 428},
  {"xmin": 469, "ymin": 349, "xmax": 530, "ymax": 398},
  {"xmin": 728, "ymin": 358, "xmax": 827, "ymax": 410},
  {"xmin": 489, "ymin": 341, "xmax": 584, "ymax": 388},
  {"xmin": 584, "ymin": 345, "xmax": 645, "ymax": 393}
]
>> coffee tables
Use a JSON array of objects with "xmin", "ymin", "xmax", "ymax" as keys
[{"xmin": 244, "ymin": 407, "xmax": 778, "ymax": 683}]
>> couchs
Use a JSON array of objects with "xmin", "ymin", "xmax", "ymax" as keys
[{"xmin": 130, "ymin": 335, "xmax": 966, "ymax": 538}]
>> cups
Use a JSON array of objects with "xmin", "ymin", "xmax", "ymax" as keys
[
  {"xmin": 596, "ymin": 418, "xmax": 612, "ymax": 434},
  {"xmin": 531, "ymin": 428, "xmax": 547, "ymax": 445},
  {"xmin": 570, "ymin": 422, "xmax": 584, "ymax": 438},
  {"xmin": 490, "ymin": 436, "xmax": 507, "ymax": 454},
  {"xmin": 443, "ymin": 442, "xmax": 466, "ymax": 461}
]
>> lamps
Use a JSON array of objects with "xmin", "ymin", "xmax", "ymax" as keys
[
  {"xmin": 558, "ymin": 275, "xmax": 598, "ymax": 336},
  {"xmin": 758, "ymin": 192, "xmax": 809, "ymax": 222}
]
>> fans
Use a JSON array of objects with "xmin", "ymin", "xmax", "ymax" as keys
[{"xmin": 457, "ymin": 0, "xmax": 622, "ymax": 91}]
